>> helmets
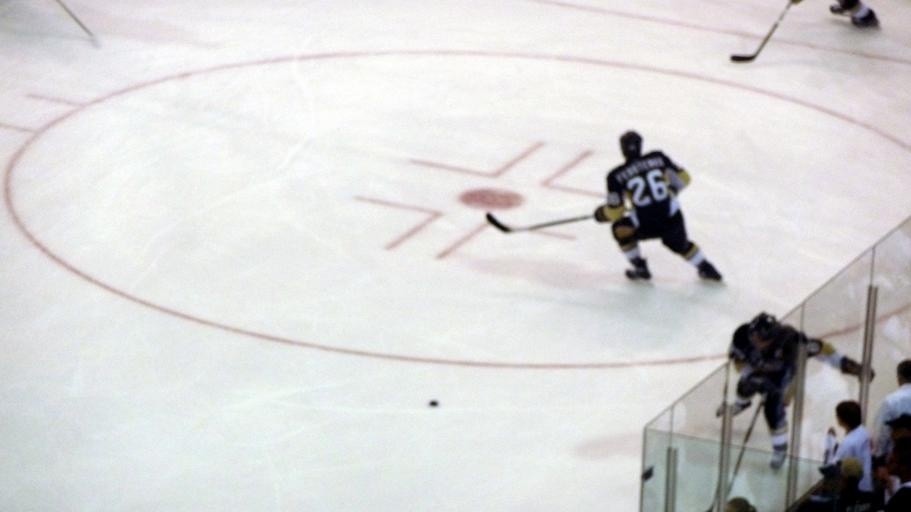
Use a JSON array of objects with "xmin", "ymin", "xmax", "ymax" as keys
[
  {"xmin": 748, "ymin": 313, "xmax": 780, "ymax": 339},
  {"xmin": 619, "ymin": 131, "xmax": 643, "ymax": 156}
]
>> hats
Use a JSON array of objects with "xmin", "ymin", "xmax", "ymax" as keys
[
  {"xmin": 820, "ymin": 456, "xmax": 864, "ymax": 481},
  {"xmin": 883, "ymin": 412, "xmax": 911, "ymax": 433}
]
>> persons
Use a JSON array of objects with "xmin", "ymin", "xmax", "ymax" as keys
[
  {"xmin": 790, "ymin": 0, "xmax": 879, "ymax": 27},
  {"xmin": 718, "ymin": 358, "xmax": 911, "ymax": 512},
  {"xmin": 595, "ymin": 132, "xmax": 723, "ymax": 281},
  {"xmin": 718, "ymin": 314, "xmax": 875, "ymax": 470}
]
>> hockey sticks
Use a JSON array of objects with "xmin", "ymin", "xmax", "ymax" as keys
[
  {"xmin": 731, "ymin": 0, "xmax": 797, "ymax": 62},
  {"xmin": 487, "ymin": 211, "xmax": 597, "ymax": 232}
]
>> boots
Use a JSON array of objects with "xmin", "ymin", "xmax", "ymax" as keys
[
  {"xmin": 829, "ymin": 3, "xmax": 878, "ymax": 27},
  {"xmin": 625, "ymin": 260, "xmax": 650, "ymax": 280},
  {"xmin": 731, "ymin": 400, "xmax": 751, "ymax": 416},
  {"xmin": 768, "ymin": 444, "xmax": 787, "ymax": 471},
  {"xmin": 695, "ymin": 261, "xmax": 722, "ymax": 282}
]
